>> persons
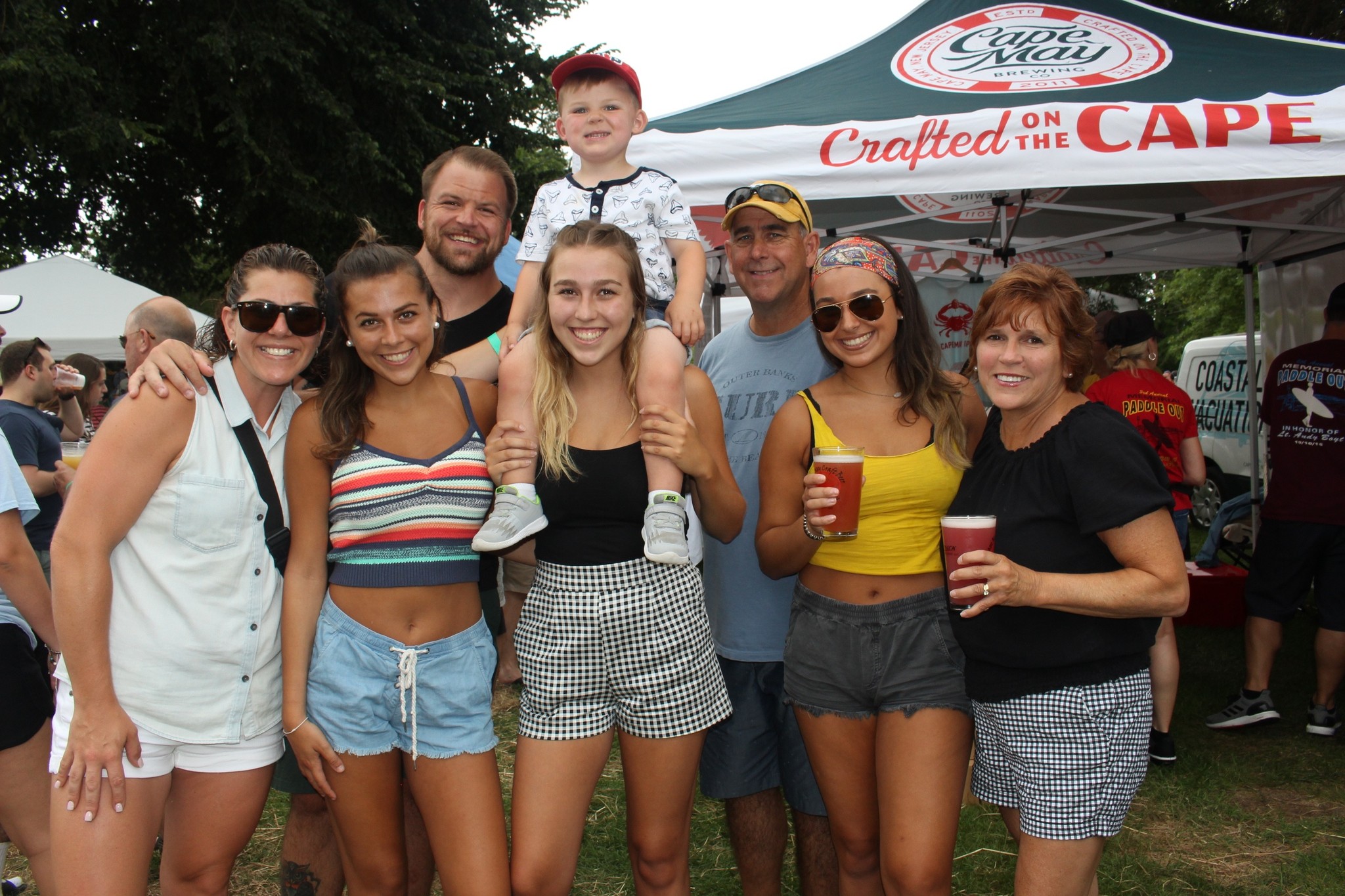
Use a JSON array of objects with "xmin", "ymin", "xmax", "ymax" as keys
[
  {"xmin": 751, "ymin": 234, "xmax": 973, "ymax": 896},
  {"xmin": 1203, "ymin": 282, "xmax": 1345, "ymax": 735},
  {"xmin": 471, "ymin": 53, "xmax": 708, "ymax": 567},
  {"xmin": 42, "ymin": 241, "xmax": 328, "ymax": 896},
  {"xmin": 480, "ymin": 219, "xmax": 746, "ymax": 895},
  {"xmin": 279, "ymin": 215, "xmax": 512, "ymax": 896},
  {"xmin": 938, "ymin": 262, "xmax": 1206, "ymax": 896},
  {"xmin": 686, "ymin": 180, "xmax": 846, "ymax": 896},
  {"xmin": 274, "ymin": 140, "xmax": 519, "ymax": 896},
  {"xmin": 0, "ymin": 293, "xmax": 195, "ymax": 896}
]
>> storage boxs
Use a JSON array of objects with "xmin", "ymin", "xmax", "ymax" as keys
[{"xmin": 1170, "ymin": 561, "xmax": 1249, "ymax": 629}]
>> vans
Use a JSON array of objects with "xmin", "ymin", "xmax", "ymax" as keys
[{"xmin": 1176, "ymin": 334, "xmax": 1269, "ymax": 532}]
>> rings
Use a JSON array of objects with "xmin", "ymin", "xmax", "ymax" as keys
[{"xmin": 984, "ymin": 582, "xmax": 989, "ymax": 596}]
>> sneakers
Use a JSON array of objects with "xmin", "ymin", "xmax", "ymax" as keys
[
  {"xmin": 640, "ymin": 493, "xmax": 690, "ymax": 565},
  {"xmin": 470, "ymin": 486, "xmax": 549, "ymax": 552},
  {"xmin": 1305, "ymin": 701, "xmax": 1342, "ymax": 735},
  {"xmin": 1204, "ymin": 687, "xmax": 1282, "ymax": 729}
]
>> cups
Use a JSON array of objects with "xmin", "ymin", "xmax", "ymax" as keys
[
  {"xmin": 59, "ymin": 442, "xmax": 89, "ymax": 471},
  {"xmin": 940, "ymin": 515, "xmax": 997, "ymax": 612},
  {"xmin": 812, "ymin": 446, "xmax": 865, "ymax": 536},
  {"xmin": 53, "ymin": 365, "xmax": 85, "ymax": 390}
]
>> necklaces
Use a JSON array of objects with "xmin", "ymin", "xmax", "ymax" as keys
[{"xmin": 840, "ymin": 372, "xmax": 903, "ymax": 399}]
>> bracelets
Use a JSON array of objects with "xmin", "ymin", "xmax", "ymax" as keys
[
  {"xmin": 802, "ymin": 515, "xmax": 825, "ymax": 540},
  {"xmin": 486, "ymin": 332, "xmax": 501, "ymax": 356},
  {"xmin": 282, "ymin": 717, "xmax": 307, "ymax": 735},
  {"xmin": 58, "ymin": 391, "xmax": 75, "ymax": 401},
  {"xmin": 45, "ymin": 644, "xmax": 61, "ymax": 666},
  {"xmin": 65, "ymin": 481, "xmax": 73, "ymax": 490}
]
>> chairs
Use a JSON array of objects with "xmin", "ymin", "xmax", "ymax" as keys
[{"xmin": 1215, "ymin": 486, "xmax": 1322, "ymax": 623}]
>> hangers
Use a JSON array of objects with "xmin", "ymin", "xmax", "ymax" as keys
[{"xmin": 914, "ymin": 249, "xmax": 992, "ymax": 286}]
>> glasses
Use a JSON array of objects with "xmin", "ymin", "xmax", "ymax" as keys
[
  {"xmin": 810, "ymin": 294, "xmax": 893, "ymax": 334},
  {"xmin": 24, "ymin": 336, "xmax": 45, "ymax": 367},
  {"xmin": 724, "ymin": 184, "xmax": 813, "ymax": 233},
  {"xmin": 231, "ymin": 301, "xmax": 326, "ymax": 338},
  {"xmin": 118, "ymin": 329, "xmax": 156, "ymax": 349}
]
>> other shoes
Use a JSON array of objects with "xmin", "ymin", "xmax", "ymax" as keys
[{"xmin": 1149, "ymin": 732, "xmax": 1177, "ymax": 765}]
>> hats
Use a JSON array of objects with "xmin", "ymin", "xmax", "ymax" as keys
[
  {"xmin": 1103, "ymin": 310, "xmax": 1166, "ymax": 348},
  {"xmin": 721, "ymin": 179, "xmax": 814, "ymax": 235},
  {"xmin": 0, "ymin": 294, "xmax": 23, "ymax": 315},
  {"xmin": 551, "ymin": 52, "xmax": 643, "ymax": 111}
]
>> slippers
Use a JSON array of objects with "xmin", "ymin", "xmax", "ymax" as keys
[{"xmin": 2, "ymin": 876, "xmax": 25, "ymax": 896}]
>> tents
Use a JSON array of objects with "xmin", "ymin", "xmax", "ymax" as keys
[
  {"xmin": 0, "ymin": 257, "xmax": 217, "ymax": 362},
  {"xmin": 563, "ymin": 0, "xmax": 1345, "ymax": 551}
]
